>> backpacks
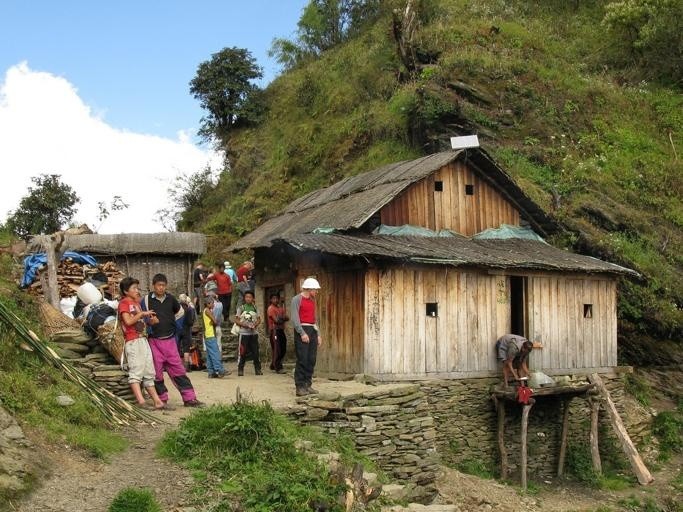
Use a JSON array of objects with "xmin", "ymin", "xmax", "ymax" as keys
[{"xmin": 189, "ymin": 346, "xmax": 206, "ymax": 370}]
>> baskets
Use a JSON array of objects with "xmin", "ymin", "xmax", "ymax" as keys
[{"xmin": 96, "ymin": 318, "xmax": 129, "ymax": 364}]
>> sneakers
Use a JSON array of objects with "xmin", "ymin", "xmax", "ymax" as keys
[
  {"xmin": 238, "ymin": 368, "xmax": 243, "ymax": 376},
  {"xmin": 278, "ymin": 368, "xmax": 287, "ymax": 374},
  {"xmin": 255, "ymin": 369, "xmax": 264, "ymax": 375},
  {"xmin": 208, "ymin": 370, "xmax": 231, "ymax": 378}
]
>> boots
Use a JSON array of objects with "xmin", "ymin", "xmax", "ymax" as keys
[{"xmin": 296, "ymin": 380, "xmax": 318, "ymax": 396}]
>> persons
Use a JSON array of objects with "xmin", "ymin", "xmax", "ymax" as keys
[
  {"xmin": 495, "ymin": 334, "xmax": 532, "ymax": 389},
  {"xmin": 290, "ymin": 278, "xmax": 321, "ymax": 397},
  {"xmin": 116, "ymin": 258, "xmax": 289, "ymax": 411}
]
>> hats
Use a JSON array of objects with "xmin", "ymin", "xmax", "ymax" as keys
[{"xmin": 302, "ymin": 278, "xmax": 321, "ymax": 290}]
